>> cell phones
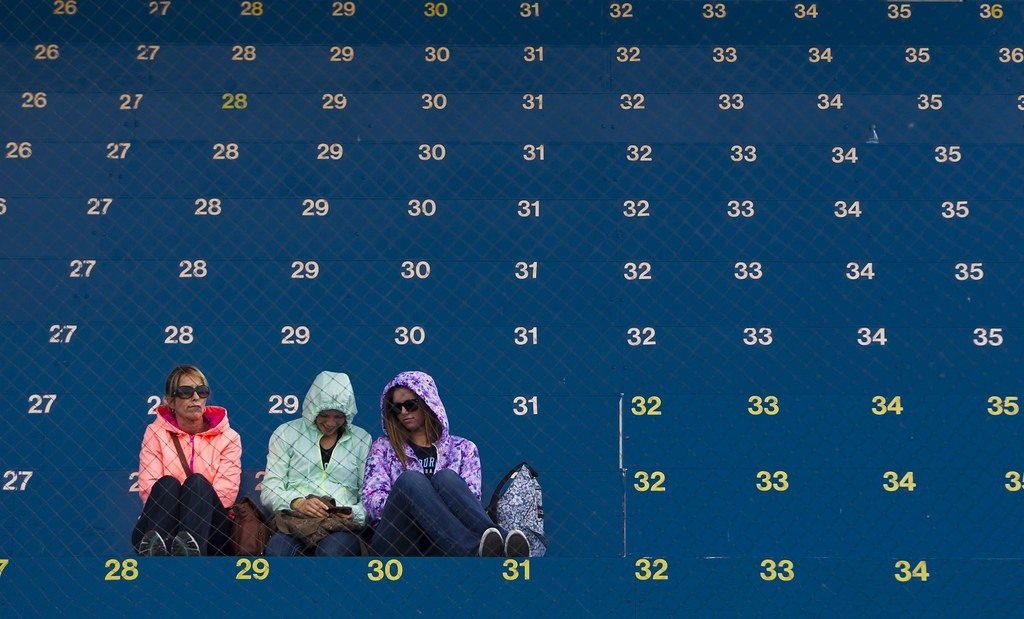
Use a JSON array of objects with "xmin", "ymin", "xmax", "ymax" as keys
[{"xmin": 323, "ymin": 507, "xmax": 352, "ymax": 518}]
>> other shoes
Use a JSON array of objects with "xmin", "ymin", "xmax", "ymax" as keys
[
  {"xmin": 479, "ymin": 528, "xmax": 504, "ymax": 557},
  {"xmin": 171, "ymin": 530, "xmax": 200, "ymax": 556},
  {"xmin": 139, "ymin": 530, "xmax": 167, "ymax": 556},
  {"xmin": 504, "ymin": 529, "xmax": 531, "ymax": 558}
]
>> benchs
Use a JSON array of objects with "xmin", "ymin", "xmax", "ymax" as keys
[{"xmin": 0, "ymin": 0, "xmax": 1024, "ymax": 619}]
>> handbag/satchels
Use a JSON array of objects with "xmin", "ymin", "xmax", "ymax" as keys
[
  {"xmin": 275, "ymin": 493, "xmax": 354, "ymax": 547},
  {"xmin": 231, "ymin": 496, "xmax": 270, "ymax": 555}
]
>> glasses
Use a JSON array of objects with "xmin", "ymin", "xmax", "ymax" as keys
[
  {"xmin": 391, "ymin": 397, "xmax": 421, "ymax": 414},
  {"xmin": 171, "ymin": 385, "xmax": 210, "ymax": 399}
]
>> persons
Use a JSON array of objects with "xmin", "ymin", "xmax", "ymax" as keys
[
  {"xmin": 132, "ymin": 365, "xmax": 243, "ymax": 556},
  {"xmin": 261, "ymin": 370, "xmax": 373, "ymax": 558},
  {"xmin": 364, "ymin": 371, "xmax": 531, "ymax": 558}
]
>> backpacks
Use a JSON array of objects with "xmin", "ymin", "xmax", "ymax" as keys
[{"xmin": 487, "ymin": 462, "xmax": 547, "ymax": 557}]
